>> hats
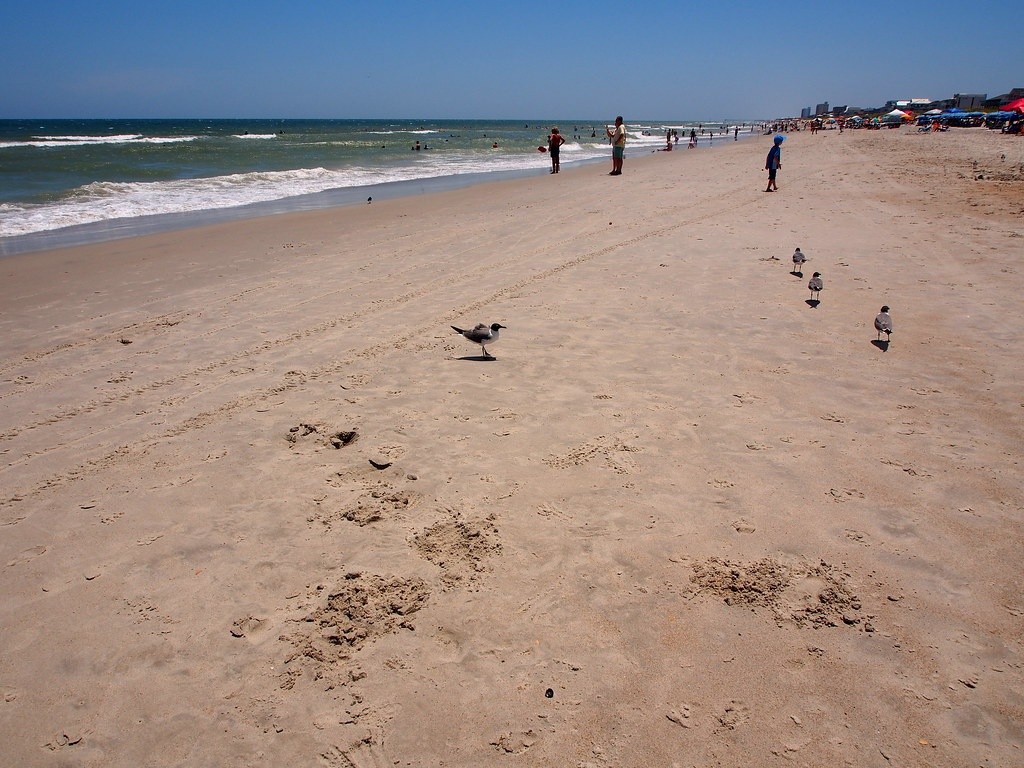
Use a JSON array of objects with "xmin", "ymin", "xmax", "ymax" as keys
[{"xmin": 774, "ymin": 134, "xmax": 787, "ymax": 144}]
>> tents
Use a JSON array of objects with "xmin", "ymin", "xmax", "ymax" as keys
[{"xmin": 812, "ymin": 97, "xmax": 1024, "ymax": 124}]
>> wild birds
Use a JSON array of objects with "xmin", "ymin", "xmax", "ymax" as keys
[
  {"xmin": 808, "ymin": 272, "xmax": 824, "ymax": 302},
  {"xmin": 792, "ymin": 248, "xmax": 807, "ymax": 274},
  {"xmin": 874, "ymin": 306, "xmax": 894, "ymax": 343},
  {"xmin": 450, "ymin": 323, "xmax": 507, "ymax": 360},
  {"xmin": 367, "ymin": 196, "xmax": 373, "ymax": 204}
]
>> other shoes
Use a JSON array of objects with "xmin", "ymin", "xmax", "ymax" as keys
[
  {"xmin": 550, "ymin": 171, "xmax": 555, "ymax": 174},
  {"xmin": 609, "ymin": 170, "xmax": 621, "ymax": 175},
  {"xmin": 556, "ymin": 172, "xmax": 558, "ymax": 173},
  {"xmin": 774, "ymin": 186, "xmax": 780, "ymax": 190},
  {"xmin": 766, "ymin": 188, "xmax": 774, "ymax": 192}
]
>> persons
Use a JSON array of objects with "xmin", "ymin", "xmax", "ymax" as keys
[
  {"xmin": 765, "ymin": 134, "xmax": 786, "ymax": 192},
  {"xmin": 244, "ymin": 116, "xmax": 1024, "ymax": 175}
]
